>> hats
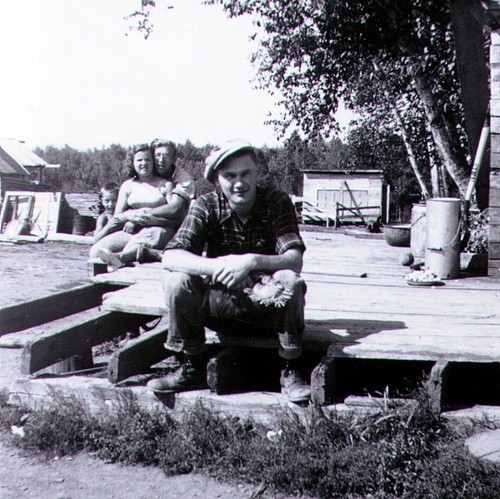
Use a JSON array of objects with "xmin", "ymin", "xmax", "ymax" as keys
[{"xmin": 204, "ymin": 137, "xmax": 258, "ymax": 185}]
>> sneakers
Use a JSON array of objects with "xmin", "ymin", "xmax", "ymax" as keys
[
  {"xmin": 147, "ymin": 361, "xmax": 208, "ymax": 392},
  {"xmin": 280, "ymin": 367, "xmax": 312, "ymax": 402}
]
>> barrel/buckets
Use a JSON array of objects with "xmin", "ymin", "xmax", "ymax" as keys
[
  {"xmin": 411, "ymin": 202, "xmax": 428, "ymax": 257},
  {"xmin": 72, "ymin": 216, "xmax": 93, "ymax": 236},
  {"xmin": 425, "ymin": 198, "xmax": 461, "ymax": 280},
  {"xmin": 384, "ymin": 224, "xmax": 410, "ymax": 247}
]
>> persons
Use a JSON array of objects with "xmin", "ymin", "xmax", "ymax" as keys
[
  {"xmin": 89, "ymin": 140, "xmax": 196, "ymax": 267},
  {"xmin": 145, "ymin": 139, "xmax": 311, "ymax": 402}
]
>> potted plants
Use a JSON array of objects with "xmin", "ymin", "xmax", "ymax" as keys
[{"xmin": 458, "ymin": 209, "xmax": 489, "ymax": 272}]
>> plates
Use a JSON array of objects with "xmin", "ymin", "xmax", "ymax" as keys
[{"xmin": 406, "ymin": 279, "xmax": 439, "ymax": 286}]
